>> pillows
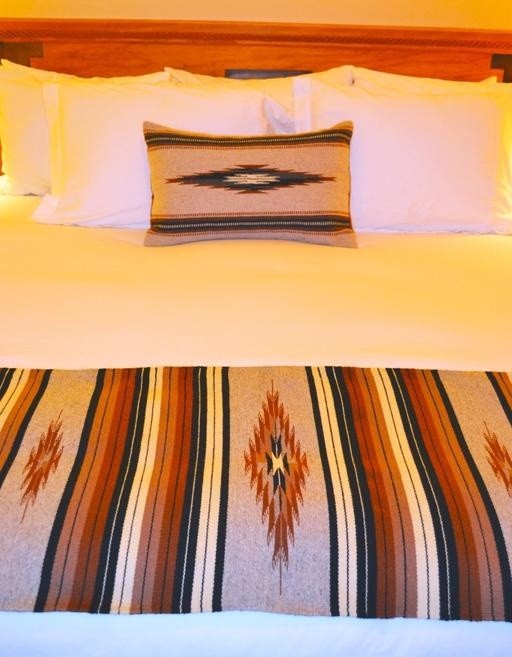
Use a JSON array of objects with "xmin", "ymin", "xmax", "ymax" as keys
[
  {"xmin": 160, "ymin": 62, "xmax": 359, "ymax": 98},
  {"xmin": 285, "ymin": 74, "xmax": 506, "ymax": 241},
  {"xmin": 0, "ymin": 54, "xmax": 178, "ymax": 197},
  {"xmin": 134, "ymin": 112, "xmax": 362, "ymax": 252},
  {"xmin": 337, "ymin": 63, "xmax": 512, "ymax": 238},
  {"xmin": 20, "ymin": 69, "xmax": 330, "ymax": 238}
]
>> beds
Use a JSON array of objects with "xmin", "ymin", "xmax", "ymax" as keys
[{"xmin": 0, "ymin": 15, "xmax": 511, "ymax": 657}]
